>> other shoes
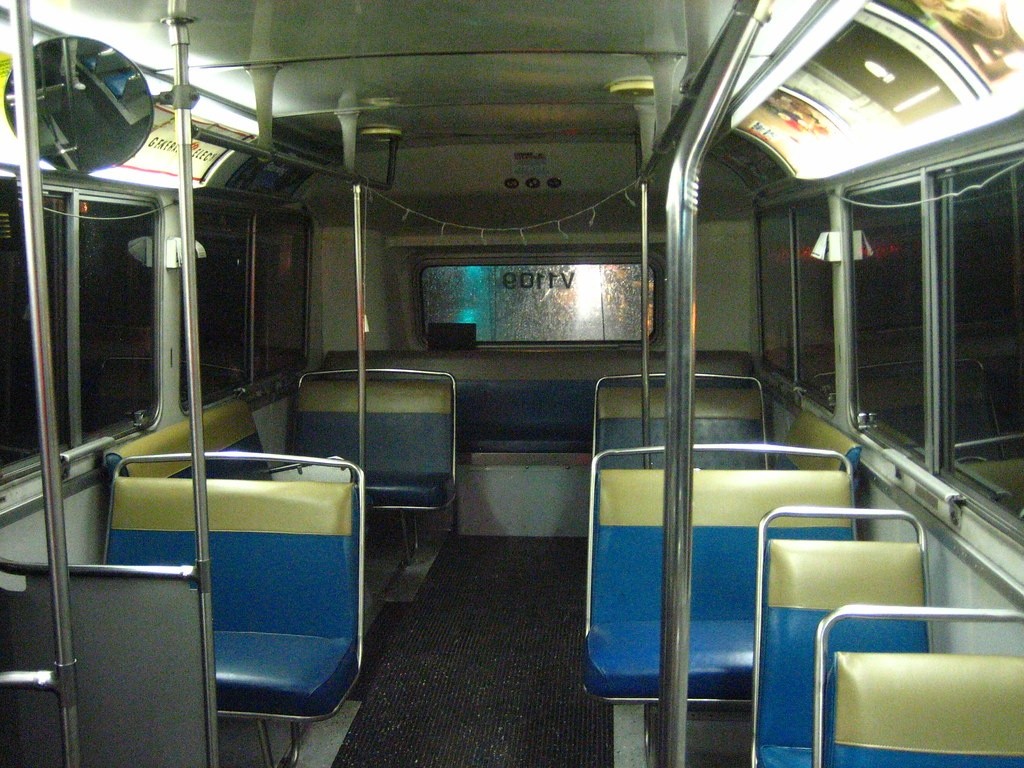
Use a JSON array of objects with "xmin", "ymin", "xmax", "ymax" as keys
[{"xmin": 74, "ymin": 82, "xmax": 86, "ymax": 90}]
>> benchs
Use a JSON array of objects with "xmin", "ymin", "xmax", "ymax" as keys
[{"xmin": 88, "ymin": 351, "xmax": 1022, "ymax": 766}]
